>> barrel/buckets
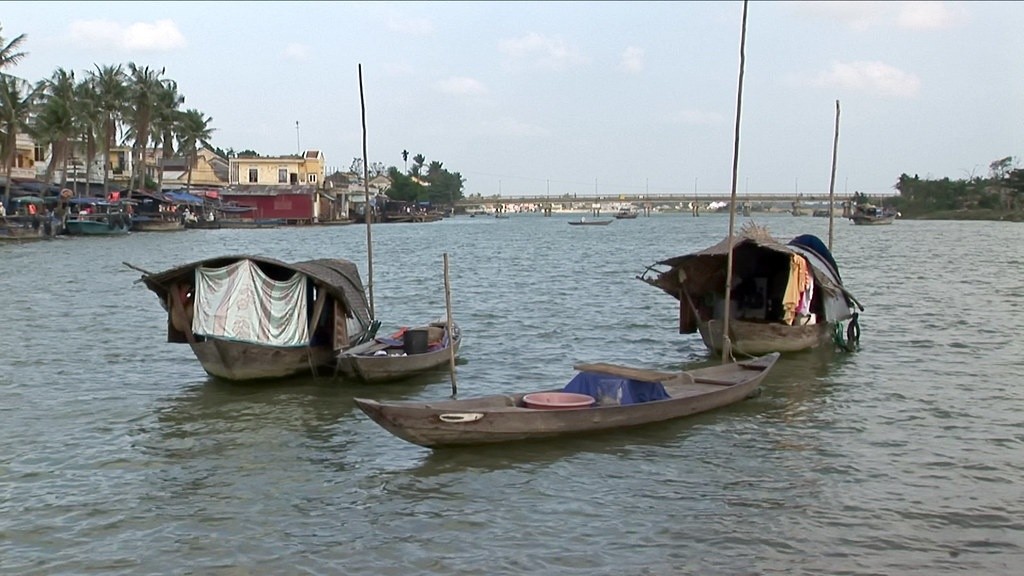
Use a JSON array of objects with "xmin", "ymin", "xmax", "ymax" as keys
[{"xmin": 403, "ymin": 330, "xmax": 429, "ymax": 355}]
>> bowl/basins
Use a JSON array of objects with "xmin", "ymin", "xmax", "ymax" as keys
[{"xmin": 522, "ymin": 392, "xmax": 596, "ymax": 409}]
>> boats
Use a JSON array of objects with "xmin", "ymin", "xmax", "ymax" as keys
[
  {"xmin": 567, "ymin": 216, "xmax": 613, "ymax": 225},
  {"xmin": 133, "ymin": 252, "xmax": 374, "ymax": 385},
  {"xmin": 0, "ymin": 184, "xmax": 282, "ymax": 241},
  {"xmin": 848, "ymin": 209, "xmax": 898, "ymax": 225},
  {"xmin": 334, "ymin": 308, "xmax": 464, "ymax": 383},
  {"xmin": 350, "ymin": 2, "xmax": 781, "ymax": 451},
  {"xmin": 616, "ymin": 210, "xmax": 638, "ymax": 219},
  {"xmin": 682, "ymin": 96, "xmax": 843, "ymax": 355}
]
